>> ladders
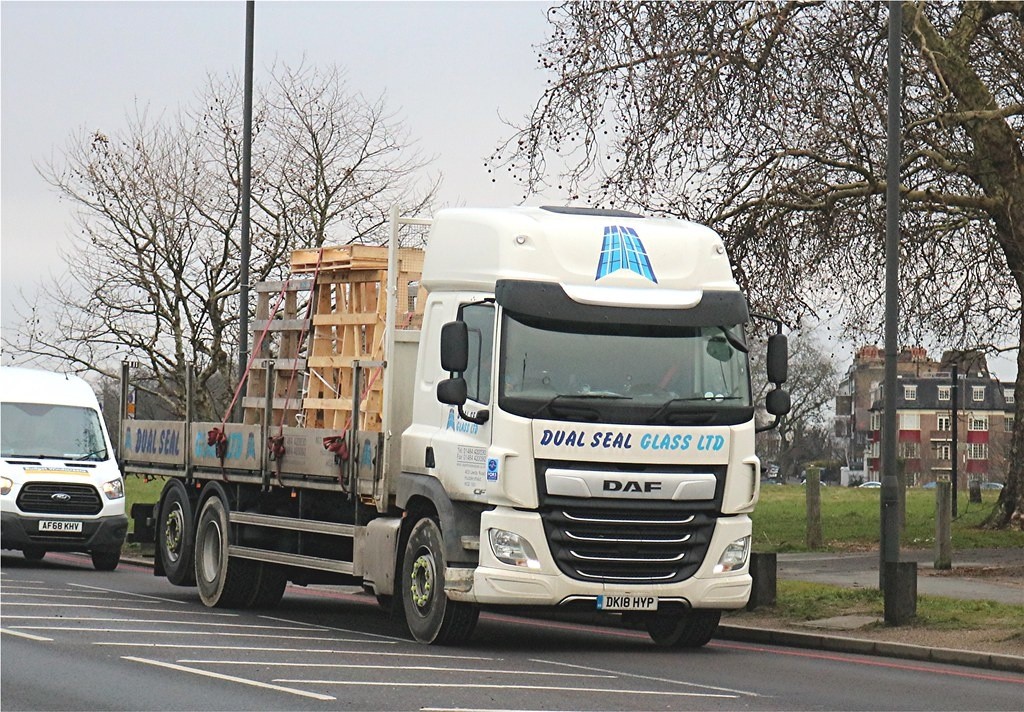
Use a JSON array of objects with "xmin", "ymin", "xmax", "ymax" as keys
[
  {"xmin": 301, "ymin": 266, "xmax": 390, "ymax": 432},
  {"xmin": 242, "ymin": 277, "xmax": 318, "ymax": 428}
]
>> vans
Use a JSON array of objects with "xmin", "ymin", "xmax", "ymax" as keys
[{"xmin": 0, "ymin": 366, "xmax": 129, "ymax": 567}]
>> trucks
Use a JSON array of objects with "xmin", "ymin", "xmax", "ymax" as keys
[{"xmin": 120, "ymin": 205, "xmax": 794, "ymax": 650}]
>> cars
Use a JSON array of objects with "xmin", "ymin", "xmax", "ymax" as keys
[
  {"xmin": 977, "ymin": 482, "xmax": 1004, "ymax": 490},
  {"xmin": 798, "ymin": 479, "xmax": 827, "ymax": 487},
  {"xmin": 853, "ymin": 481, "xmax": 882, "ymax": 490},
  {"xmin": 921, "ymin": 481, "xmax": 954, "ymax": 491}
]
